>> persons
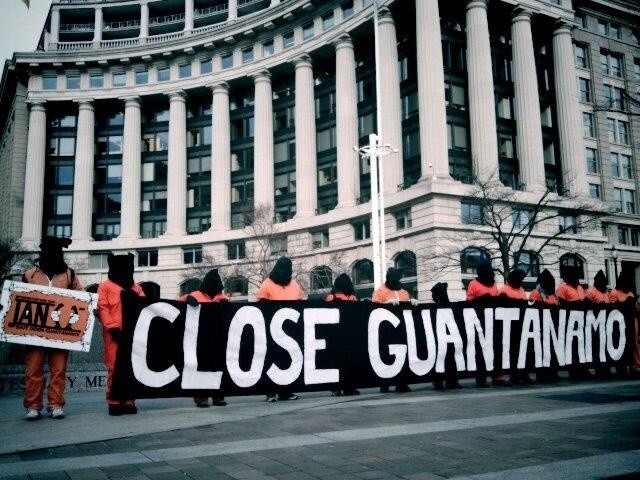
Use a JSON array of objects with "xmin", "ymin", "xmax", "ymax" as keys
[
  {"xmin": 325, "ymin": 271, "xmax": 360, "ymax": 396},
  {"xmin": 467, "ymin": 259, "xmax": 640, "ymax": 388},
  {"xmin": 430, "ymin": 283, "xmax": 463, "ymax": 391},
  {"xmin": 20, "ymin": 236, "xmax": 85, "ymax": 420},
  {"xmin": 97, "ymin": 252, "xmax": 147, "ymax": 416},
  {"xmin": 256, "ymin": 254, "xmax": 306, "ymax": 402},
  {"xmin": 372, "ymin": 265, "xmax": 413, "ymax": 392},
  {"xmin": 180, "ymin": 268, "xmax": 231, "ymax": 406}
]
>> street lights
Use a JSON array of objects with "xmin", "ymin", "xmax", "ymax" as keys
[
  {"xmin": 609, "ymin": 246, "xmax": 619, "ymax": 289},
  {"xmin": 353, "ymin": 134, "xmax": 399, "ymax": 291}
]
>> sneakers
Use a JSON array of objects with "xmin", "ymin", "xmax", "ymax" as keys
[
  {"xmin": 379, "ymin": 386, "xmax": 389, "ymax": 393},
  {"xmin": 445, "ymin": 381, "xmax": 461, "ymax": 388},
  {"xmin": 27, "ymin": 410, "xmax": 41, "ymax": 418},
  {"xmin": 212, "ymin": 399, "xmax": 227, "ymax": 405},
  {"xmin": 395, "ymin": 385, "xmax": 411, "ymax": 391},
  {"xmin": 477, "ymin": 378, "xmax": 528, "ymax": 389},
  {"xmin": 109, "ymin": 405, "xmax": 138, "ymax": 416},
  {"xmin": 432, "ymin": 383, "xmax": 444, "ymax": 390},
  {"xmin": 266, "ymin": 394, "xmax": 278, "ymax": 402},
  {"xmin": 197, "ymin": 400, "xmax": 209, "ymax": 407},
  {"xmin": 280, "ymin": 392, "xmax": 302, "ymax": 400},
  {"xmin": 333, "ymin": 388, "xmax": 362, "ymax": 396},
  {"xmin": 48, "ymin": 408, "xmax": 65, "ymax": 419}
]
